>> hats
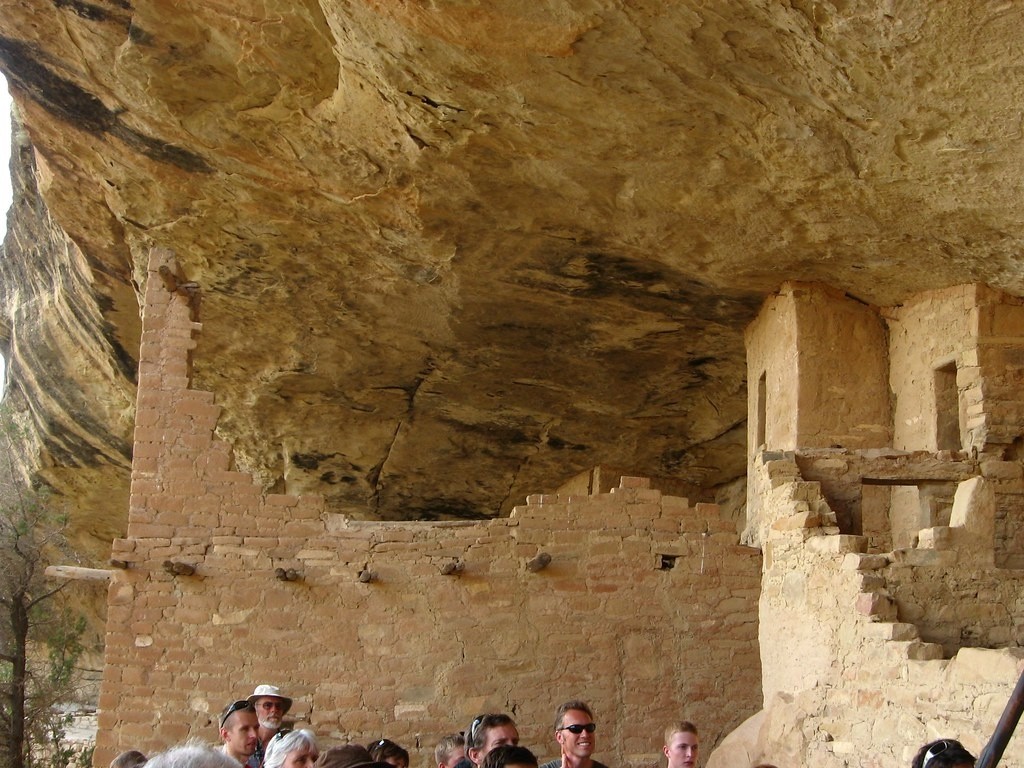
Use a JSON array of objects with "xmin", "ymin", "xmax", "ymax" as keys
[
  {"xmin": 246, "ymin": 684, "xmax": 293, "ymax": 715},
  {"xmin": 312, "ymin": 744, "xmax": 380, "ymax": 768},
  {"xmin": 109, "ymin": 751, "xmax": 148, "ymax": 768}
]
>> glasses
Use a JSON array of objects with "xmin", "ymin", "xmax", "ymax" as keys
[
  {"xmin": 275, "ymin": 729, "xmax": 291, "ymax": 748},
  {"xmin": 558, "ymin": 723, "xmax": 596, "ymax": 734},
  {"xmin": 472, "ymin": 713, "xmax": 494, "ymax": 742},
  {"xmin": 221, "ymin": 700, "xmax": 250, "ymax": 728},
  {"xmin": 922, "ymin": 741, "xmax": 963, "ymax": 768},
  {"xmin": 373, "ymin": 739, "xmax": 391, "ymax": 753}
]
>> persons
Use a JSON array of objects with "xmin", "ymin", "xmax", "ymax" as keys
[
  {"xmin": 262, "ymin": 728, "xmax": 318, "ymax": 768},
  {"xmin": 216, "ymin": 699, "xmax": 260, "ymax": 768},
  {"xmin": 144, "ymin": 737, "xmax": 243, "ymax": 768},
  {"xmin": 368, "ymin": 739, "xmax": 409, "ymax": 768},
  {"xmin": 435, "ymin": 731, "xmax": 467, "ymax": 768},
  {"xmin": 245, "ymin": 685, "xmax": 293, "ymax": 768},
  {"xmin": 110, "ymin": 751, "xmax": 147, "ymax": 768},
  {"xmin": 313, "ymin": 743, "xmax": 396, "ymax": 768},
  {"xmin": 912, "ymin": 739, "xmax": 977, "ymax": 768},
  {"xmin": 464, "ymin": 713, "xmax": 519, "ymax": 768},
  {"xmin": 540, "ymin": 700, "xmax": 610, "ymax": 768},
  {"xmin": 480, "ymin": 746, "xmax": 539, "ymax": 768},
  {"xmin": 663, "ymin": 721, "xmax": 699, "ymax": 768}
]
